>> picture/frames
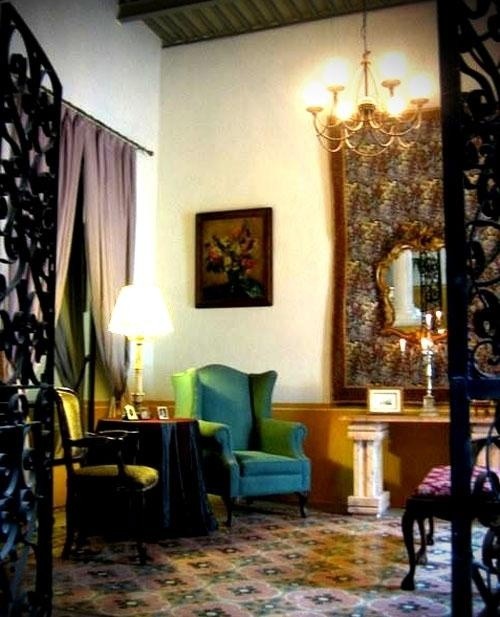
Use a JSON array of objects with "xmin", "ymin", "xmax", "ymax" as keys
[
  {"xmin": 195, "ymin": 207, "xmax": 274, "ymax": 309},
  {"xmin": 366, "ymin": 386, "xmax": 404, "ymax": 415},
  {"xmin": 122, "ymin": 403, "xmax": 138, "ymax": 420},
  {"xmin": 156, "ymin": 405, "xmax": 170, "ymax": 420},
  {"xmin": 138, "ymin": 406, "xmax": 151, "ymax": 420}
]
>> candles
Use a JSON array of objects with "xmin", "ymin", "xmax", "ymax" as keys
[{"xmin": 424, "ymin": 313, "xmax": 433, "ymax": 350}]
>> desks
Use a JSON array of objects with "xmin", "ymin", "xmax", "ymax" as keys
[
  {"xmin": 84, "ymin": 418, "xmax": 220, "ymax": 534},
  {"xmin": 337, "ymin": 414, "xmax": 500, "ymax": 519}
]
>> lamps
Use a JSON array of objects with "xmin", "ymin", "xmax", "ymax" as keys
[
  {"xmin": 108, "ymin": 285, "xmax": 172, "ymax": 419},
  {"xmin": 304, "ymin": 55, "xmax": 431, "ymax": 157}
]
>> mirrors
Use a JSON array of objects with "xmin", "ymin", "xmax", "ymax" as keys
[{"xmin": 370, "ymin": 221, "xmax": 448, "ymax": 344}]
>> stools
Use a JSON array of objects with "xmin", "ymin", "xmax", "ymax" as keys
[{"xmin": 400, "ymin": 465, "xmax": 500, "ymax": 591}]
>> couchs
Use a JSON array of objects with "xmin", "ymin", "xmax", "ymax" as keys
[{"xmin": 171, "ymin": 363, "xmax": 312, "ymax": 527}]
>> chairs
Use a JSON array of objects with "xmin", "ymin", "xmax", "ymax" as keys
[{"xmin": 54, "ymin": 387, "xmax": 160, "ymax": 567}]
